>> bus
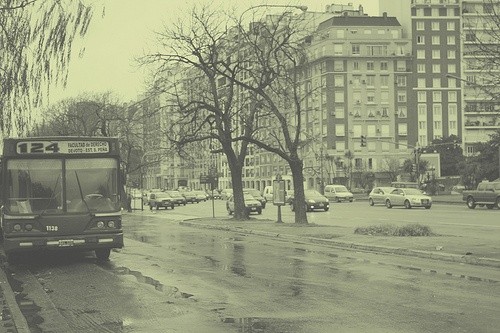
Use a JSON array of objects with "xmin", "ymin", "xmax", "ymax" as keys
[{"xmin": 0, "ymin": 136, "xmax": 128, "ymax": 267}]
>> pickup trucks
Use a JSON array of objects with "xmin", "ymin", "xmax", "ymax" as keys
[{"xmin": 462, "ymin": 181, "xmax": 500, "ymax": 210}]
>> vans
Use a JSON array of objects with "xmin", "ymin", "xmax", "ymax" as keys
[{"xmin": 391, "ymin": 182, "xmax": 419, "ymax": 189}]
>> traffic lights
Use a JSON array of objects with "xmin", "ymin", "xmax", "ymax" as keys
[{"xmin": 360, "ymin": 135, "xmax": 367, "ymax": 147}]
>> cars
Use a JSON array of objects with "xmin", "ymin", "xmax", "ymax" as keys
[
  {"xmin": 243, "ymin": 189, "xmax": 267, "ymax": 209},
  {"xmin": 383, "ymin": 187, "xmax": 432, "ymax": 209},
  {"xmin": 226, "ymin": 192, "xmax": 262, "ymax": 215},
  {"xmin": 164, "ymin": 190, "xmax": 186, "ymax": 206},
  {"xmin": 149, "ymin": 192, "xmax": 174, "ymax": 210},
  {"xmin": 263, "ymin": 186, "xmax": 291, "ymax": 203},
  {"xmin": 221, "ymin": 189, "xmax": 233, "ymax": 201},
  {"xmin": 289, "ymin": 189, "xmax": 330, "ymax": 212},
  {"xmin": 368, "ymin": 187, "xmax": 396, "ymax": 206},
  {"xmin": 127, "ymin": 186, "xmax": 221, "ymax": 206}
]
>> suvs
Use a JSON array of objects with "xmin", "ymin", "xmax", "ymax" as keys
[{"xmin": 324, "ymin": 185, "xmax": 353, "ymax": 203}]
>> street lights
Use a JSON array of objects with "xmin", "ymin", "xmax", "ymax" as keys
[{"xmin": 235, "ymin": 5, "xmax": 309, "ymax": 157}]
